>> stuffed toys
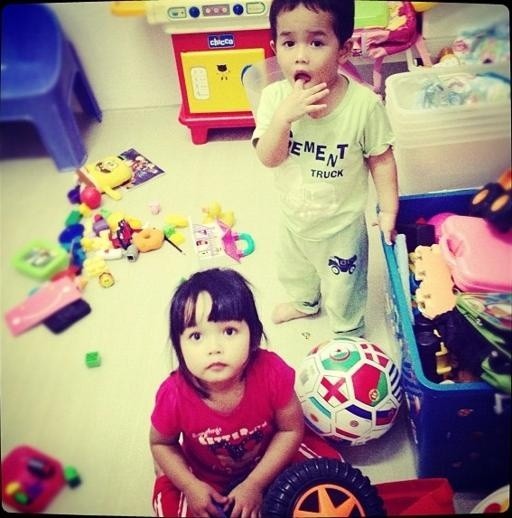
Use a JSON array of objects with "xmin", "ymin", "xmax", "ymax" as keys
[{"xmin": 466, "ymin": 168, "xmax": 511, "ymax": 235}]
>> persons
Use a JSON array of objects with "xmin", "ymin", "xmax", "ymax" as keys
[
  {"xmin": 150, "ymin": 268, "xmax": 347, "ymax": 518},
  {"xmin": 249, "ymin": 1, "xmax": 402, "ymax": 349}
]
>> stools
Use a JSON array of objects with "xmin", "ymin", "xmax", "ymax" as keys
[{"xmin": 0, "ymin": 4, "xmax": 103, "ymax": 175}]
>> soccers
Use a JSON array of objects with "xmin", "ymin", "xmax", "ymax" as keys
[{"xmin": 295, "ymin": 333, "xmax": 403, "ymax": 445}]
